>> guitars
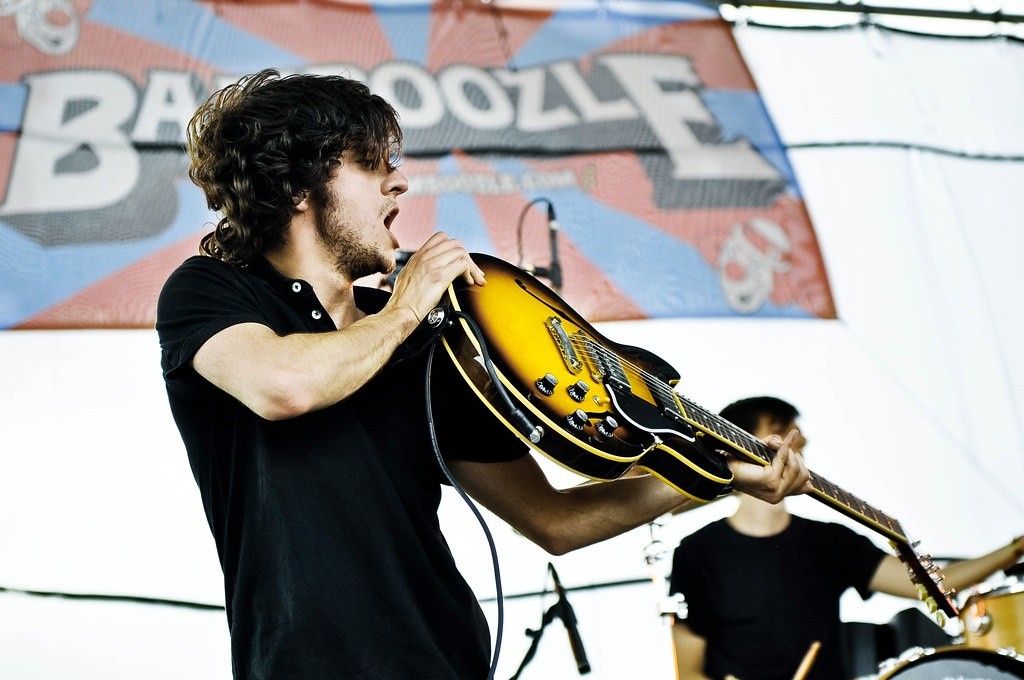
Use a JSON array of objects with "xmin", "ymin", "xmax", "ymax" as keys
[{"xmin": 427, "ymin": 252, "xmax": 964, "ymax": 637}]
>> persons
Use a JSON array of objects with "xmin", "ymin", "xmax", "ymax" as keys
[
  {"xmin": 155, "ymin": 74, "xmax": 813, "ymax": 680},
  {"xmin": 669, "ymin": 396, "xmax": 1024, "ymax": 680}
]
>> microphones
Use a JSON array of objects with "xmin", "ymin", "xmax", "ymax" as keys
[
  {"xmin": 548, "ymin": 201, "xmax": 561, "ymax": 291},
  {"xmin": 549, "ymin": 561, "xmax": 590, "ymax": 674}
]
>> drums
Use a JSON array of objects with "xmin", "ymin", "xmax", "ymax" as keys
[
  {"xmin": 878, "ymin": 642, "xmax": 1024, "ymax": 680},
  {"xmin": 957, "ymin": 584, "xmax": 1024, "ymax": 655}
]
